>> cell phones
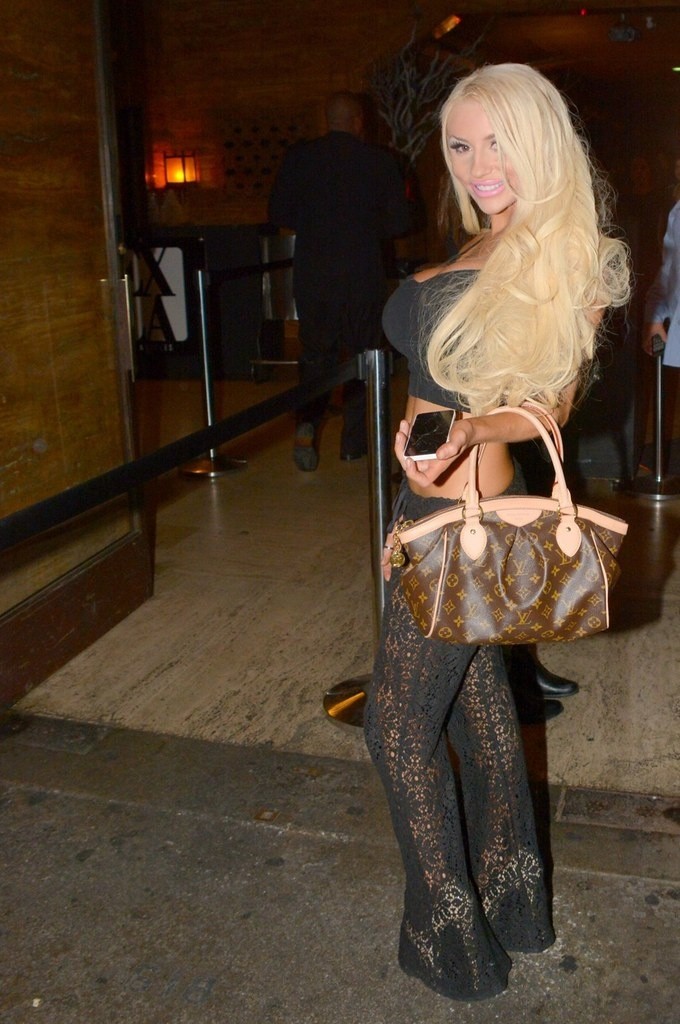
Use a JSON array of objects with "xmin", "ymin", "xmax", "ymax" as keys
[{"xmin": 403, "ymin": 410, "xmax": 457, "ymax": 461}]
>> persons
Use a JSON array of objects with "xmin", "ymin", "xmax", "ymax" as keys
[
  {"xmin": 642, "ymin": 197, "xmax": 680, "ymax": 366},
  {"xmin": 267, "ymin": 92, "xmax": 408, "ymax": 472},
  {"xmin": 362, "ymin": 63, "xmax": 631, "ymax": 1002}
]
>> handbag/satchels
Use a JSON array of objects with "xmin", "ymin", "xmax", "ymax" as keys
[{"xmin": 396, "ymin": 402, "xmax": 630, "ymax": 644}]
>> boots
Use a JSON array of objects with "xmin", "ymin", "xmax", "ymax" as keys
[
  {"xmin": 533, "ymin": 656, "xmax": 580, "ymax": 698},
  {"xmin": 500, "ymin": 658, "xmax": 564, "ymax": 723}
]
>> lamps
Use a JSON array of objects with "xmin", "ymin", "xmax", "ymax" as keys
[{"xmin": 163, "ymin": 147, "xmax": 200, "ymax": 190}]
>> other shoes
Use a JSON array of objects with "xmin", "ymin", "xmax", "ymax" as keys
[
  {"xmin": 340, "ymin": 445, "xmax": 370, "ymax": 461},
  {"xmin": 292, "ymin": 421, "xmax": 320, "ymax": 471}
]
